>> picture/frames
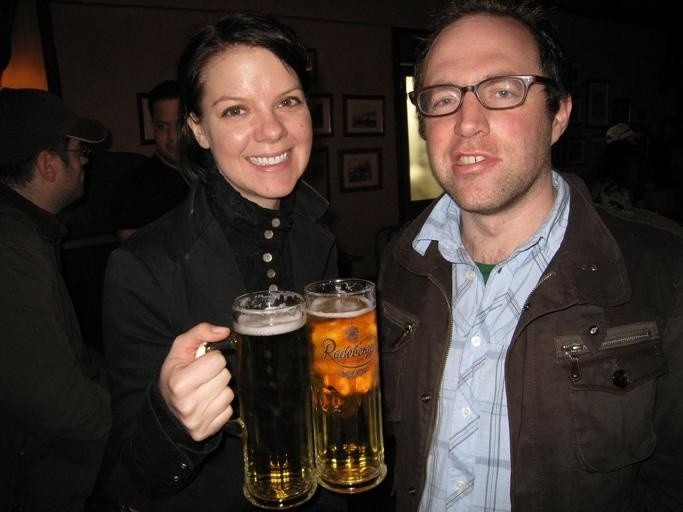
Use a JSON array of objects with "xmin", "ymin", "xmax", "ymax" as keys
[{"xmin": 309, "ymin": 93, "xmax": 387, "ymax": 193}]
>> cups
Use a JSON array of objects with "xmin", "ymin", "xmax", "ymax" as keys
[
  {"xmin": 192, "ymin": 289, "xmax": 318, "ymax": 512},
  {"xmin": 306, "ymin": 275, "xmax": 388, "ymax": 496}
]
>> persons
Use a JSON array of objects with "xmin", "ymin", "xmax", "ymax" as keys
[
  {"xmin": 100, "ymin": 6, "xmax": 349, "ymax": 511},
  {"xmin": 375, "ymin": 2, "xmax": 682, "ymax": 511},
  {"xmin": 601, "ymin": 122, "xmax": 644, "ymax": 199},
  {"xmin": 0, "ymin": 86, "xmax": 122, "ymax": 512},
  {"xmin": 106, "ymin": 80, "xmax": 196, "ymax": 242}
]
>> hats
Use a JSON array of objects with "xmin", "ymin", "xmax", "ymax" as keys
[
  {"xmin": 605, "ymin": 124, "xmax": 642, "ymax": 144},
  {"xmin": 0, "ymin": 88, "xmax": 109, "ymax": 161}
]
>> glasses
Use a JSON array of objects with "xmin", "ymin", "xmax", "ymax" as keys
[
  {"xmin": 50, "ymin": 145, "xmax": 91, "ymax": 156},
  {"xmin": 408, "ymin": 76, "xmax": 558, "ymax": 117}
]
